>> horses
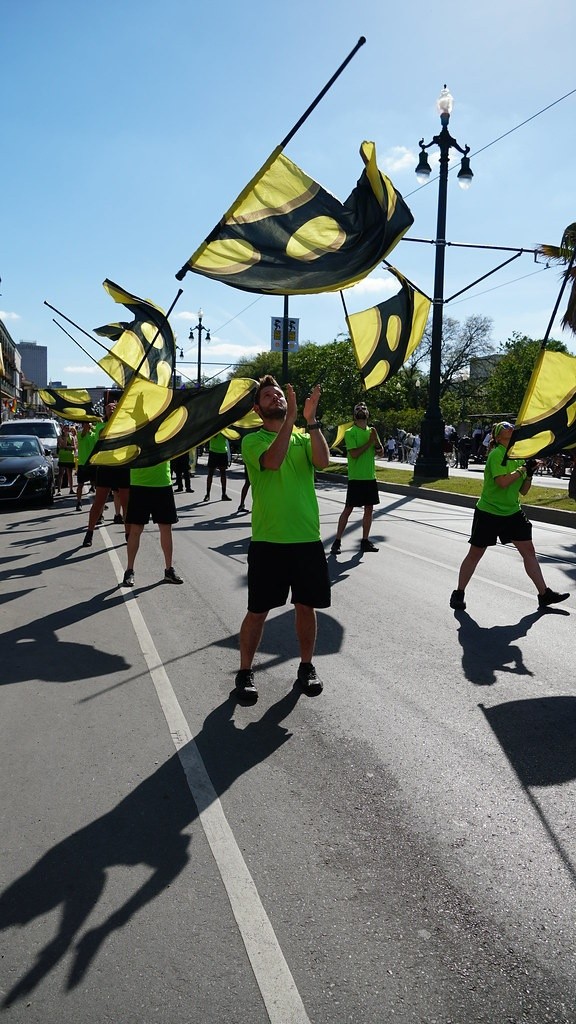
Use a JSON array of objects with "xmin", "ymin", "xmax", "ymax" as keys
[{"xmin": 397, "ymin": 428, "xmax": 416, "ymax": 463}]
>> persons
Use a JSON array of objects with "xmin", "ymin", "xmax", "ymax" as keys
[
  {"xmin": 175, "ymin": 451, "xmax": 196, "ymax": 493},
  {"xmin": 81, "ymin": 468, "xmax": 132, "ymax": 547},
  {"xmin": 53, "ymin": 421, "xmax": 108, "ymax": 512},
  {"xmin": 234, "ymin": 374, "xmax": 330, "ymax": 700},
  {"xmin": 330, "ymin": 401, "xmax": 384, "ymax": 555},
  {"xmin": 120, "ymin": 460, "xmax": 188, "ymax": 587},
  {"xmin": 448, "ymin": 420, "xmax": 570, "ymax": 612},
  {"xmin": 238, "ymin": 462, "xmax": 253, "ymax": 513},
  {"xmin": 382, "ymin": 422, "xmax": 497, "ymax": 468},
  {"xmin": 203, "ymin": 430, "xmax": 233, "ymax": 503}
]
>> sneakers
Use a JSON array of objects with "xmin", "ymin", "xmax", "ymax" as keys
[
  {"xmin": 235, "ymin": 671, "xmax": 259, "ymax": 699},
  {"xmin": 297, "ymin": 662, "xmax": 324, "ymax": 691},
  {"xmin": 331, "ymin": 540, "xmax": 341, "ymax": 554},
  {"xmin": 449, "ymin": 589, "xmax": 467, "ymax": 610},
  {"xmin": 360, "ymin": 538, "xmax": 380, "ymax": 552},
  {"xmin": 164, "ymin": 567, "xmax": 183, "ymax": 584},
  {"xmin": 123, "ymin": 572, "xmax": 135, "ymax": 587},
  {"xmin": 537, "ymin": 587, "xmax": 570, "ymax": 607}
]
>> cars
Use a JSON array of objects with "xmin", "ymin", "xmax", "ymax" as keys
[{"xmin": 0, "ymin": 435, "xmax": 60, "ymax": 505}]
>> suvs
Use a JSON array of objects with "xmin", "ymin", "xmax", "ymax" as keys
[{"xmin": 0, "ymin": 419, "xmax": 65, "ymax": 481}]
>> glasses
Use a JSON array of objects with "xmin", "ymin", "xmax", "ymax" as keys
[{"xmin": 498, "ymin": 423, "xmax": 515, "ymax": 437}]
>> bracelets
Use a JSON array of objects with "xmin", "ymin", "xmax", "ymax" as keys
[
  {"xmin": 375, "ymin": 445, "xmax": 382, "ymax": 453},
  {"xmin": 525, "ymin": 477, "xmax": 532, "ymax": 481},
  {"xmin": 516, "ymin": 468, "xmax": 525, "ymax": 477},
  {"xmin": 307, "ymin": 419, "xmax": 321, "ymax": 430}
]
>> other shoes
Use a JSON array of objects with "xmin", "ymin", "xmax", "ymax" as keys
[
  {"xmin": 76, "ymin": 502, "xmax": 82, "ymax": 511},
  {"xmin": 238, "ymin": 505, "xmax": 249, "ymax": 512},
  {"xmin": 174, "ymin": 487, "xmax": 183, "ymax": 492},
  {"xmin": 114, "ymin": 514, "xmax": 125, "ymax": 524},
  {"xmin": 173, "ymin": 480, "xmax": 179, "ymax": 485},
  {"xmin": 83, "ymin": 530, "xmax": 93, "ymax": 547},
  {"xmin": 96, "ymin": 516, "xmax": 104, "ymax": 525},
  {"xmin": 222, "ymin": 495, "xmax": 231, "ymax": 501},
  {"xmin": 55, "ymin": 482, "xmax": 114, "ymax": 499},
  {"xmin": 204, "ymin": 495, "xmax": 210, "ymax": 501},
  {"xmin": 104, "ymin": 506, "xmax": 108, "ymax": 510},
  {"xmin": 186, "ymin": 488, "xmax": 194, "ymax": 493},
  {"xmin": 181, "ymin": 471, "xmax": 194, "ymax": 478}
]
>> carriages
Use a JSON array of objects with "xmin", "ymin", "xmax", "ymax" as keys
[{"xmin": 393, "ymin": 411, "xmax": 522, "ymax": 469}]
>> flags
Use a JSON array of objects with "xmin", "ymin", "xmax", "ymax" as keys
[
  {"xmin": 505, "ymin": 350, "xmax": 576, "ymax": 461},
  {"xmin": 92, "ymin": 278, "xmax": 177, "ymax": 390},
  {"xmin": 187, "ymin": 138, "xmax": 418, "ymax": 295},
  {"xmin": 331, "ymin": 421, "xmax": 355, "ymax": 447},
  {"xmin": 346, "ymin": 285, "xmax": 432, "ymax": 388},
  {"xmin": 39, "ymin": 387, "xmax": 103, "ymax": 421},
  {"xmin": 86, "ymin": 375, "xmax": 261, "ymax": 471}
]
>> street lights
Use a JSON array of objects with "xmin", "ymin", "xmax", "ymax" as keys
[
  {"xmin": 413, "ymin": 84, "xmax": 473, "ymax": 483},
  {"xmin": 188, "ymin": 308, "xmax": 212, "ymax": 389},
  {"xmin": 172, "ymin": 335, "xmax": 183, "ymax": 391}
]
